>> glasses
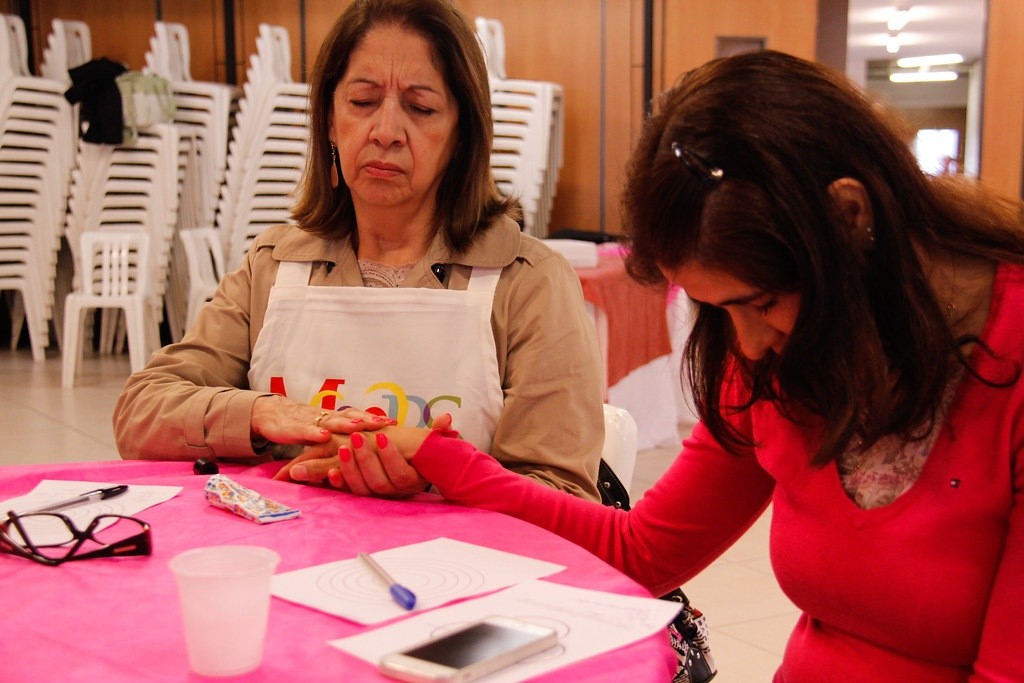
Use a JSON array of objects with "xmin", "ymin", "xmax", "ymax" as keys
[{"xmin": 0, "ymin": 510, "xmax": 153, "ymax": 567}]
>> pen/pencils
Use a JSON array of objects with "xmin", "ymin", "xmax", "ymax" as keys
[
  {"xmin": 359, "ymin": 552, "xmax": 417, "ymax": 611},
  {"xmin": 1, "ymin": 484, "xmax": 128, "ymax": 524}
]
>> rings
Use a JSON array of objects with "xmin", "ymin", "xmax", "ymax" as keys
[{"xmin": 313, "ymin": 411, "xmax": 333, "ymax": 427}]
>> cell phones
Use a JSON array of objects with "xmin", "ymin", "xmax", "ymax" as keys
[{"xmin": 380, "ymin": 615, "xmax": 557, "ymax": 683}]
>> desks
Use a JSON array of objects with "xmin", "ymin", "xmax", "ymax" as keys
[
  {"xmin": 0, "ymin": 457, "xmax": 679, "ymax": 683},
  {"xmin": 548, "ymin": 241, "xmax": 703, "ymax": 452}
]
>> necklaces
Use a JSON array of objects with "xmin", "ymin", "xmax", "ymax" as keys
[{"xmin": 945, "ymin": 256, "xmax": 958, "ymax": 323}]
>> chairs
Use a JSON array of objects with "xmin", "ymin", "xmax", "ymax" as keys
[{"xmin": 0, "ymin": 15, "xmax": 570, "ymax": 389}]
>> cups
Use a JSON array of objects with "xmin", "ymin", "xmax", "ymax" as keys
[{"xmin": 169, "ymin": 545, "xmax": 283, "ymax": 676}]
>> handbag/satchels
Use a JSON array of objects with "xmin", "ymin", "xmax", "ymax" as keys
[
  {"xmin": 118, "ymin": 74, "xmax": 176, "ymax": 144},
  {"xmin": 596, "ymin": 457, "xmax": 718, "ymax": 683}
]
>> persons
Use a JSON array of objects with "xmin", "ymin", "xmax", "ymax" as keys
[
  {"xmin": 110, "ymin": 1, "xmax": 607, "ymax": 507},
  {"xmin": 273, "ymin": 48, "xmax": 1024, "ymax": 683}
]
